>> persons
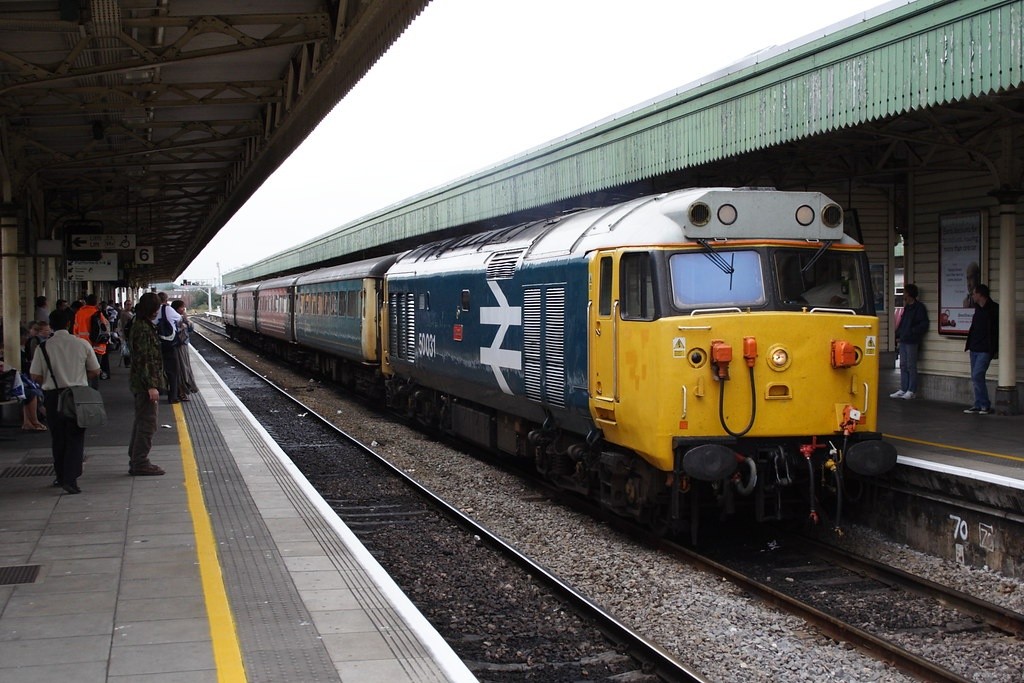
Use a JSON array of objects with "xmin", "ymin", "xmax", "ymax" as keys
[
  {"xmin": 963, "ymin": 284, "xmax": 999, "ymax": 414},
  {"xmin": 29, "ymin": 309, "xmax": 100, "ymax": 494},
  {"xmin": 889, "ymin": 284, "xmax": 929, "ymax": 398},
  {"xmin": 152, "ymin": 292, "xmax": 198, "ymax": 403},
  {"xmin": 963, "ymin": 263, "xmax": 980, "ymax": 308},
  {"xmin": 56, "ymin": 294, "xmax": 136, "ymax": 390},
  {"xmin": 0, "ymin": 296, "xmax": 49, "ymax": 430},
  {"xmin": 127, "ymin": 293, "xmax": 166, "ymax": 476}
]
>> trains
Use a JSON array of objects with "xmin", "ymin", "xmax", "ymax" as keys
[{"xmin": 219, "ymin": 187, "xmax": 898, "ymax": 553}]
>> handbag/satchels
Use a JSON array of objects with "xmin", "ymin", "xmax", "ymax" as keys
[
  {"xmin": 11, "ymin": 367, "xmax": 26, "ymax": 400},
  {"xmin": 125, "ymin": 315, "xmax": 135, "ymax": 334},
  {"xmin": 20, "ymin": 373, "xmax": 37, "ymax": 389},
  {"xmin": 57, "ymin": 385, "xmax": 108, "ymax": 428},
  {"xmin": 0, "ymin": 368, "xmax": 17, "ymax": 402},
  {"xmin": 156, "ymin": 304, "xmax": 173, "ymax": 336},
  {"xmin": 176, "ymin": 327, "xmax": 189, "ymax": 345},
  {"xmin": 121, "ymin": 339, "xmax": 130, "ymax": 357}
]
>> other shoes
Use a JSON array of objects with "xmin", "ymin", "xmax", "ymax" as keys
[
  {"xmin": 178, "ymin": 392, "xmax": 190, "ymax": 401},
  {"xmin": 100, "ymin": 374, "xmax": 110, "ymax": 380},
  {"xmin": 169, "ymin": 398, "xmax": 180, "ymax": 403},
  {"xmin": 964, "ymin": 406, "xmax": 981, "ymax": 413},
  {"xmin": 58, "ymin": 475, "xmax": 81, "ymax": 494},
  {"xmin": 127, "ymin": 463, "xmax": 166, "ymax": 476},
  {"xmin": 979, "ymin": 409, "xmax": 991, "ymax": 414}
]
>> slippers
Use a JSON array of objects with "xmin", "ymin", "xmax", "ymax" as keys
[{"xmin": 22, "ymin": 422, "xmax": 47, "ymax": 432}]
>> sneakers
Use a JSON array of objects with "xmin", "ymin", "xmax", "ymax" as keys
[
  {"xmin": 890, "ymin": 389, "xmax": 904, "ymax": 398},
  {"xmin": 903, "ymin": 390, "xmax": 916, "ymax": 400}
]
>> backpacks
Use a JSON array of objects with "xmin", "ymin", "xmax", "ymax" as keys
[
  {"xmin": 26, "ymin": 336, "xmax": 40, "ymax": 361},
  {"xmin": 90, "ymin": 310, "xmax": 110, "ymax": 343}
]
